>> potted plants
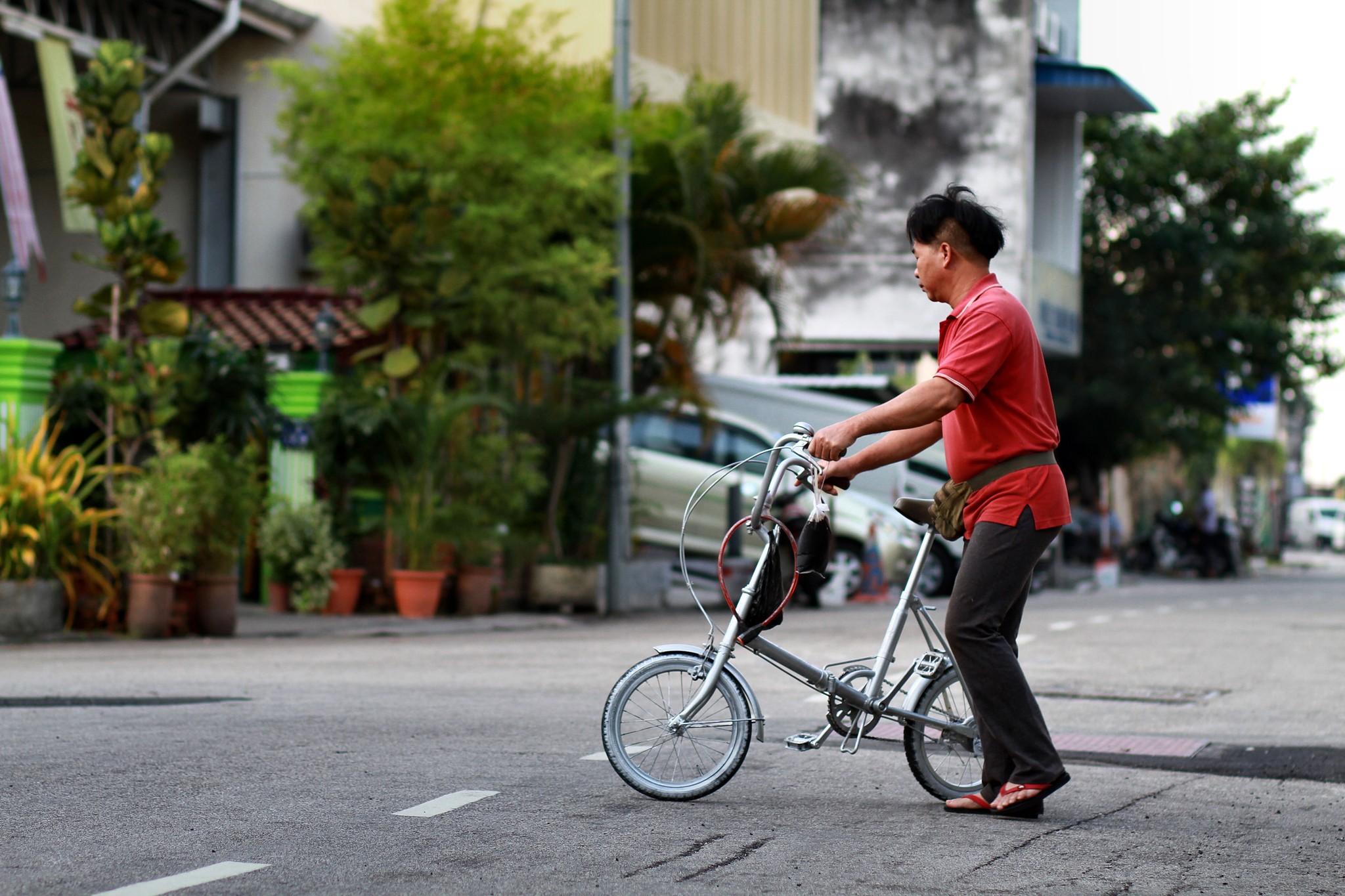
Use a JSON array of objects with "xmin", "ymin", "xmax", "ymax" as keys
[
  {"xmin": 263, "ymin": 491, "xmax": 365, "ymax": 618},
  {"xmin": 341, "ymin": 358, "xmax": 513, "ymax": 618},
  {"xmin": 452, "ymin": 511, "xmax": 508, "ymax": 616},
  {"xmin": 124, "ymin": 453, "xmax": 226, "ymax": 639},
  {"xmin": 183, "ymin": 442, "xmax": 266, "ymax": 639},
  {"xmin": 531, "ymin": 560, "xmax": 599, "ymax": 610}
]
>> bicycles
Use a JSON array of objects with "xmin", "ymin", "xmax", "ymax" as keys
[{"xmin": 601, "ymin": 421, "xmax": 982, "ymax": 800}]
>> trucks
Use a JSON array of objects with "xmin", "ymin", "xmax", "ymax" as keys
[{"xmin": 698, "ymin": 374, "xmax": 970, "ymax": 598}]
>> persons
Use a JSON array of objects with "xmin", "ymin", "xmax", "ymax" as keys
[
  {"xmin": 1194, "ymin": 483, "xmax": 1227, "ymax": 577},
  {"xmin": 813, "ymin": 181, "xmax": 1074, "ymax": 820}
]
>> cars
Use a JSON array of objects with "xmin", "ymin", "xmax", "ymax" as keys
[
  {"xmin": 596, "ymin": 393, "xmax": 921, "ymax": 602},
  {"xmin": 1063, "ymin": 474, "xmax": 1241, "ymax": 577}
]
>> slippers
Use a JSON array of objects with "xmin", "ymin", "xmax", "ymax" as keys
[
  {"xmin": 944, "ymin": 788, "xmax": 1045, "ymax": 818},
  {"xmin": 989, "ymin": 770, "xmax": 1072, "ymax": 814}
]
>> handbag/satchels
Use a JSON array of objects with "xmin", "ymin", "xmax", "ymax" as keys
[
  {"xmin": 747, "ymin": 526, "xmax": 784, "ymax": 632},
  {"xmin": 796, "ymin": 461, "xmax": 831, "ymax": 580},
  {"xmin": 928, "ymin": 479, "xmax": 977, "ymax": 541}
]
